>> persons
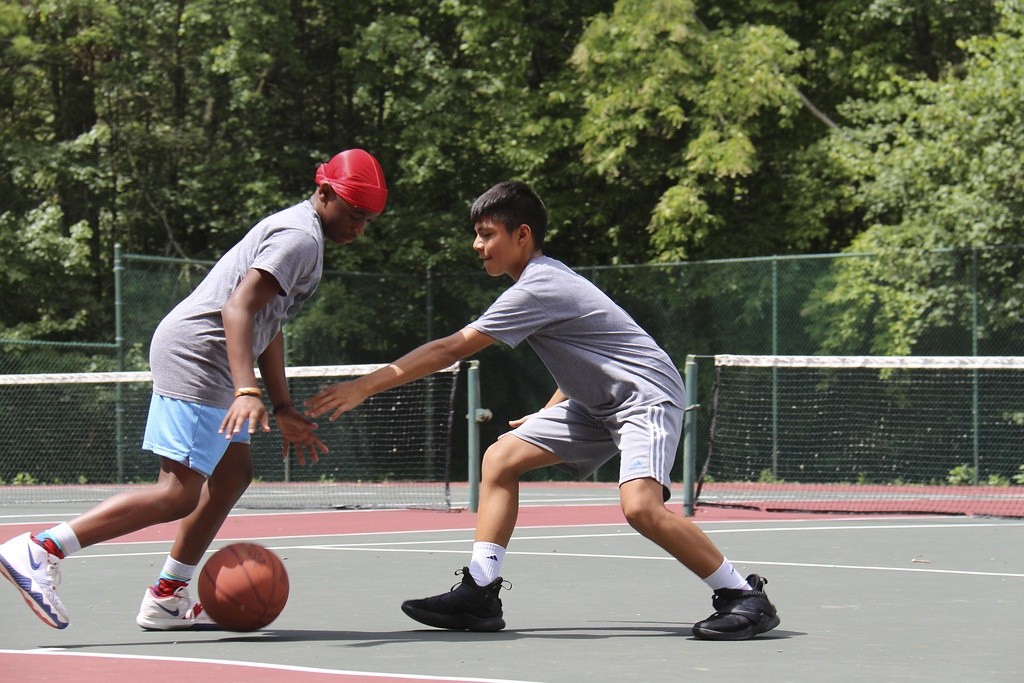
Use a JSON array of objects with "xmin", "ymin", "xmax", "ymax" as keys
[
  {"xmin": 0, "ymin": 145, "xmax": 388, "ymax": 632},
  {"xmin": 290, "ymin": 174, "xmax": 779, "ymax": 644}
]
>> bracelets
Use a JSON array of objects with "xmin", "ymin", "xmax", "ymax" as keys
[{"xmin": 232, "ymin": 387, "xmax": 264, "ymax": 397}]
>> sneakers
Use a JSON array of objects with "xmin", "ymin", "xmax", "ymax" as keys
[
  {"xmin": 400, "ymin": 566, "xmax": 512, "ymax": 633},
  {"xmin": 135, "ymin": 584, "xmax": 219, "ymax": 631},
  {"xmin": 0, "ymin": 532, "xmax": 69, "ymax": 630},
  {"xmin": 692, "ymin": 573, "xmax": 780, "ymax": 642}
]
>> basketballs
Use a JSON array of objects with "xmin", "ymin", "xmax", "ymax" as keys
[{"xmin": 197, "ymin": 543, "xmax": 289, "ymax": 630}]
